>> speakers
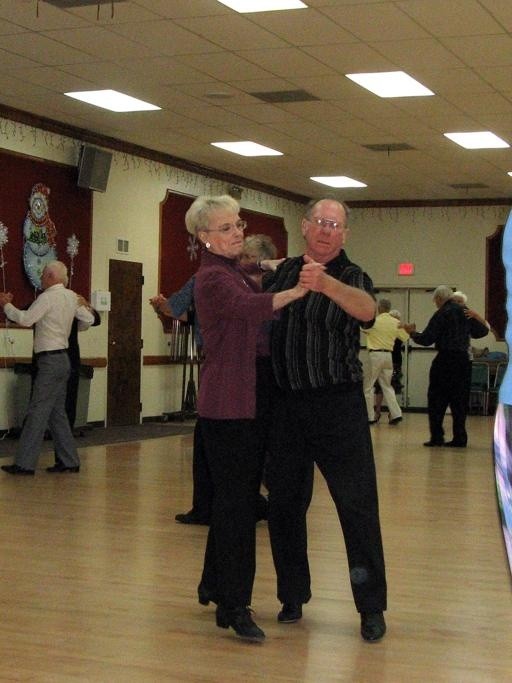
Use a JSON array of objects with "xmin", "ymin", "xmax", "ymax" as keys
[{"xmin": 77, "ymin": 145, "xmax": 113, "ymax": 192}]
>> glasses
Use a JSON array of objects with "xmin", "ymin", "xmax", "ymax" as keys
[
  {"xmin": 312, "ymin": 217, "xmax": 342, "ymax": 230},
  {"xmin": 205, "ymin": 219, "xmax": 247, "ymax": 233}
]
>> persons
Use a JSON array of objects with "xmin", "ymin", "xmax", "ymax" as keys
[
  {"xmin": 149, "ymin": 271, "xmax": 212, "ymax": 525},
  {"xmin": 264, "ymin": 195, "xmax": 395, "ymax": 642},
  {"xmin": 1, "ymin": 259, "xmax": 95, "ymax": 474},
  {"xmin": 183, "ymin": 189, "xmax": 327, "ymax": 642},
  {"xmin": 398, "ymin": 288, "xmax": 491, "ymax": 409},
  {"xmin": 1, "ymin": 290, "xmax": 101, "ymax": 436},
  {"xmin": 149, "ymin": 230, "xmax": 278, "ymax": 522},
  {"xmin": 360, "ymin": 294, "xmax": 409, "ymax": 424},
  {"xmin": 371, "ymin": 307, "xmax": 415, "ymax": 421},
  {"xmin": 398, "ymin": 285, "xmax": 491, "ymax": 448}
]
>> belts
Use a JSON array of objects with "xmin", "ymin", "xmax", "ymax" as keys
[
  {"xmin": 38, "ymin": 348, "xmax": 66, "ymax": 354},
  {"xmin": 369, "ymin": 348, "xmax": 389, "ymax": 351}
]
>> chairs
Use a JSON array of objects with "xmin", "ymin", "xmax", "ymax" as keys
[
  {"xmin": 485, "ymin": 362, "xmax": 508, "ymax": 415},
  {"xmin": 471, "ymin": 361, "xmax": 489, "ymax": 416}
]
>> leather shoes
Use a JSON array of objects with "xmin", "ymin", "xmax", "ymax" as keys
[
  {"xmin": 175, "ymin": 509, "xmax": 199, "ymax": 523},
  {"xmin": 277, "ymin": 602, "xmax": 302, "ymax": 623},
  {"xmin": 1, "ymin": 463, "xmax": 35, "ymax": 474},
  {"xmin": 361, "ymin": 610, "xmax": 386, "ymax": 640},
  {"xmin": 46, "ymin": 464, "xmax": 79, "ymax": 472},
  {"xmin": 423, "ymin": 439, "xmax": 468, "ymax": 447}
]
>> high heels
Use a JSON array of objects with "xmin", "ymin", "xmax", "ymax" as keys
[{"xmin": 215, "ymin": 600, "xmax": 265, "ymax": 640}]
